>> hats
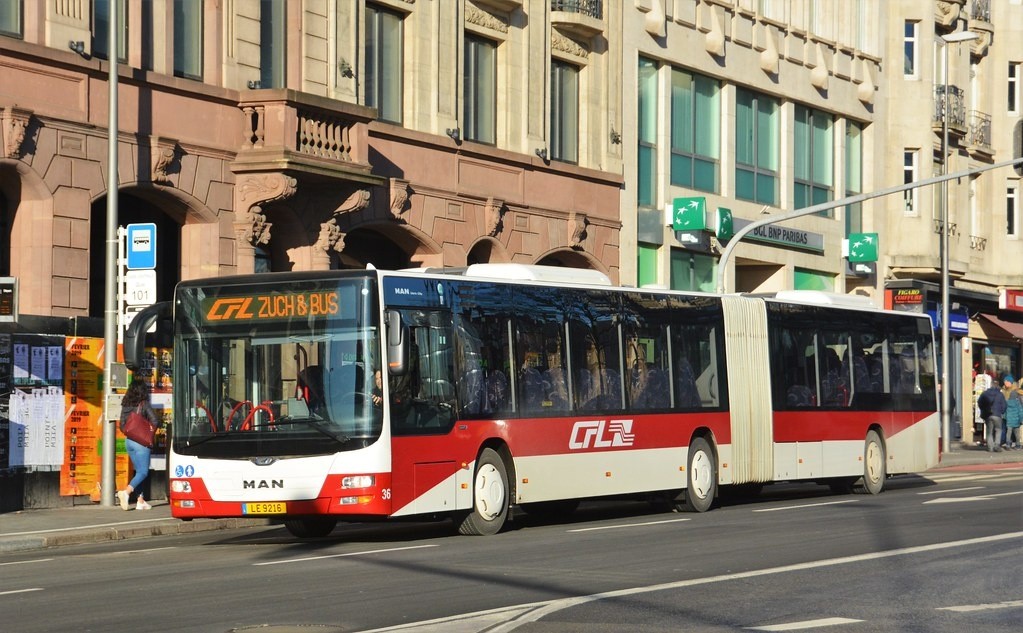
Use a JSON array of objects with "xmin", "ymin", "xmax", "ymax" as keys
[
  {"xmin": 1004, "ymin": 374, "xmax": 1014, "ymax": 384},
  {"xmin": 1009, "ymin": 391, "xmax": 1018, "ymax": 399}
]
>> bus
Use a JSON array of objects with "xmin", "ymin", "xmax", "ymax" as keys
[{"xmin": 120, "ymin": 253, "xmax": 947, "ymax": 540}]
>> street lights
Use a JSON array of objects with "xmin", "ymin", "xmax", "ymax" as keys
[{"xmin": 941, "ymin": 29, "xmax": 980, "ymax": 454}]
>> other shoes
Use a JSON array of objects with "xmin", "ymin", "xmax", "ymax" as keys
[
  {"xmin": 1015, "ymin": 445, "xmax": 1021, "ymax": 450},
  {"xmin": 118, "ymin": 490, "xmax": 130, "ymax": 510},
  {"xmin": 136, "ymin": 500, "xmax": 151, "ymax": 510},
  {"xmin": 987, "ymin": 448, "xmax": 993, "ymax": 452},
  {"xmin": 993, "ymin": 447, "xmax": 1002, "ymax": 452},
  {"xmin": 1002, "ymin": 444, "xmax": 1011, "ymax": 448}
]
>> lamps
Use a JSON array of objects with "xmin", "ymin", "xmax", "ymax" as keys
[
  {"xmin": 68, "ymin": 39, "xmax": 86, "ymax": 52},
  {"xmin": 445, "ymin": 127, "xmax": 460, "ymax": 139},
  {"xmin": 245, "ymin": 79, "xmax": 263, "ymax": 90},
  {"xmin": 535, "ymin": 148, "xmax": 548, "ymax": 159}
]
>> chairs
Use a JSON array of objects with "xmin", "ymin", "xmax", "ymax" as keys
[{"xmin": 440, "ymin": 349, "xmax": 912, "ymax": 418}]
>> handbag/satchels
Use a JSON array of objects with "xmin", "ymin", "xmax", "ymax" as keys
[
  {"xmin": 124, "ymin": 400, "xmax": 157, "ymax": 448},
  {"xmin": 980, "ymin": 403, "xmax": 991, "ymax": 420}
]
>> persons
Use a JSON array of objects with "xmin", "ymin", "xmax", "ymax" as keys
[
  {"xmin": 369, "ymin": 370, "xmax": 384, "ymax": 409},
  {"xmin": 976, "ymin": 375, "xmax": 1023, "ymax": 452},
  {"xmin": 118, "ymin": 379, "xmax": 159, "ymax": 511}
]
